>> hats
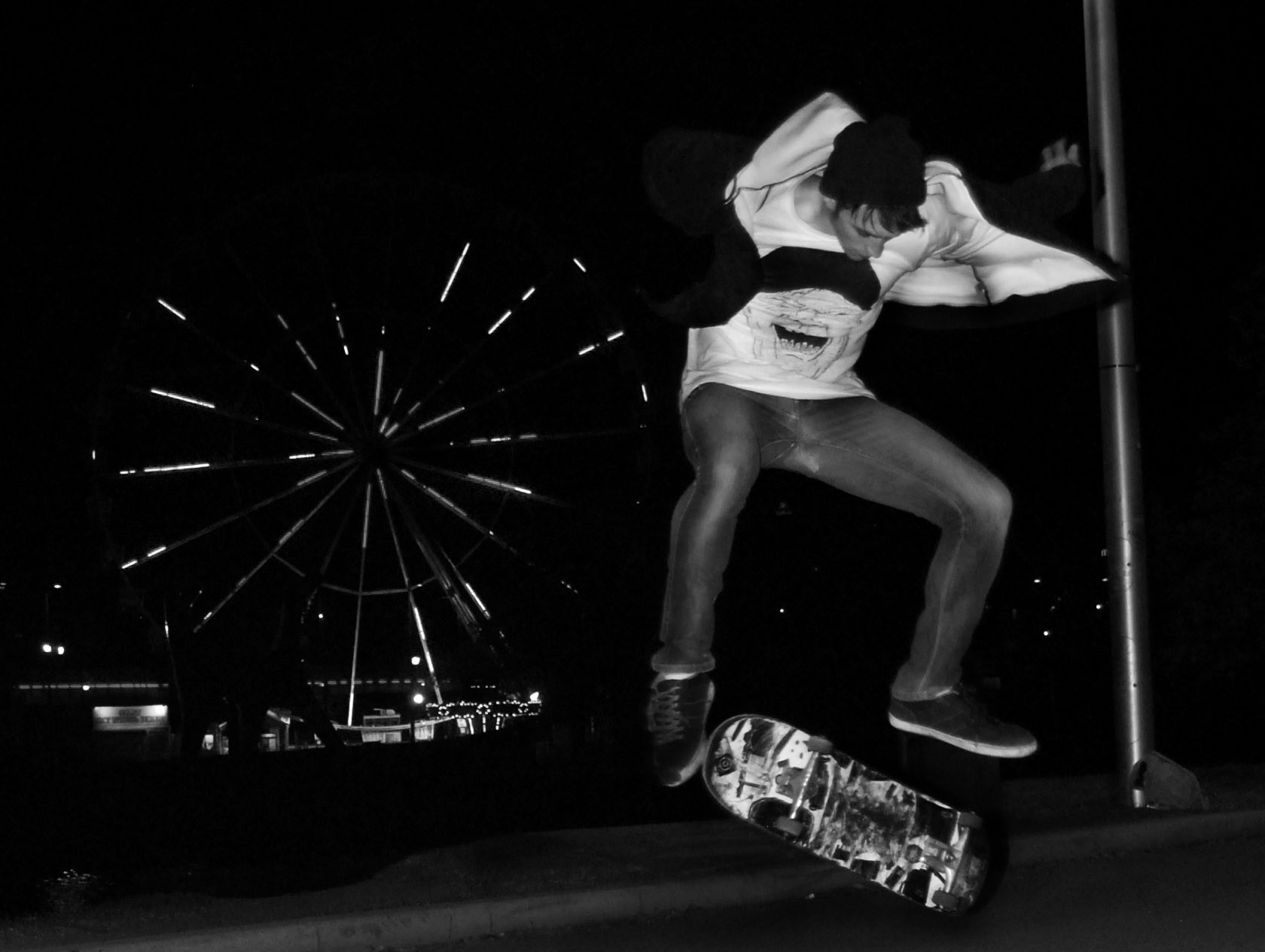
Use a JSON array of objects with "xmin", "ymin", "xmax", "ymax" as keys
[{"xmin": 819, "ymin": 120, "xmax": 928, "ymax": 208}]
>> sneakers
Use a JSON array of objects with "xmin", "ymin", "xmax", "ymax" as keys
[
  {"xmin": 887, "ymin": 687, "xmax": 1037, "ymax": 758},
  {"xmin": 646, "ymin": 669, "xmax": 716, "ymax": 786}
]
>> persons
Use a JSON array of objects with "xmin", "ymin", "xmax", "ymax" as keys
[{"xmin": 641, "ymin": 88, "xmax": 1131, "ymax": 790}]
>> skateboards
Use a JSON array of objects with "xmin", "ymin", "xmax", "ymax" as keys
[{"xmin": 701, "ymin": 714, "xmax": 991, "ymax": 920}]
